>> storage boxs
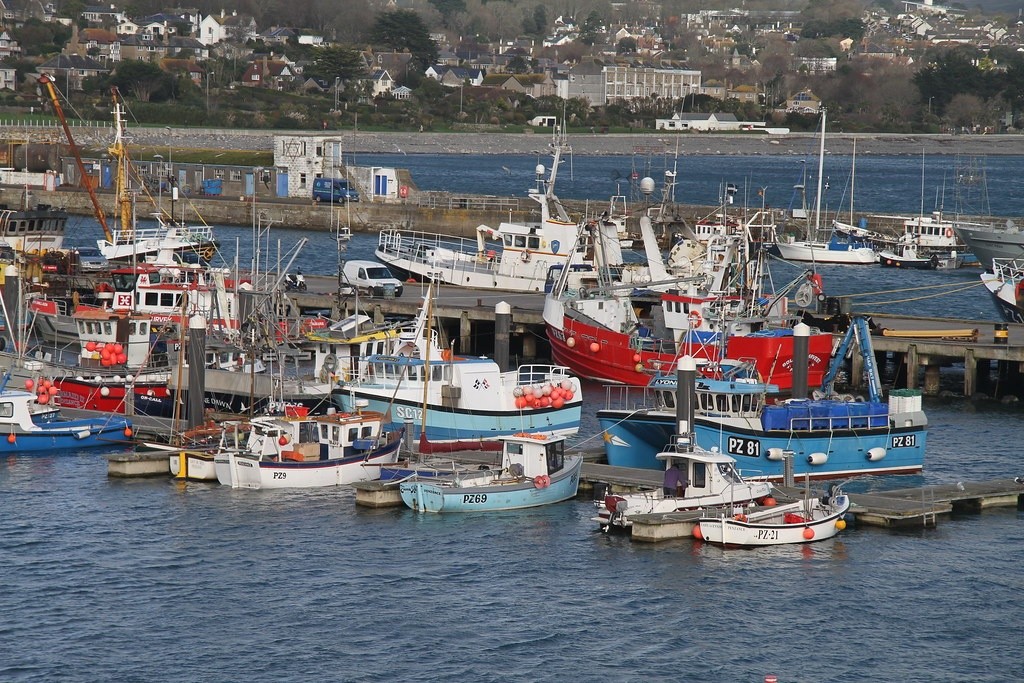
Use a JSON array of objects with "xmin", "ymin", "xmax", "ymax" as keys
[{"xmin": 293, "ymin": 442, "xmax": 321, "ymax": 462}]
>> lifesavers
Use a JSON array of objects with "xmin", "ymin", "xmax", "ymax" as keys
[
  {"xmin": 406, "ymin": 278, "xmax": 417, "ymax": 283},
  {"xmin": 734, "ymin": 514, "xmax": 747, "ymax": 523},
  {"xmin": 687, "ymin": 310, "xmax": 702, "ymax": 327},
  {"xmin": 946, "ymin": 228, "xmax": 953, "ymax": 238},
  {"xmin": 521, "ymin": 250, "xmax": 533, "ymax": 263}
]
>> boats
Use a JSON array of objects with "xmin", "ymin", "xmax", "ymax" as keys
[{"xmin": 2, "ymin": 99, "xmax": 1024, "ymax": 550}]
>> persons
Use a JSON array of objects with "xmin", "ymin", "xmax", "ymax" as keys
[{"xmin": 662, "ymin": 464, "xmax": 686, "ymax": 499}]
